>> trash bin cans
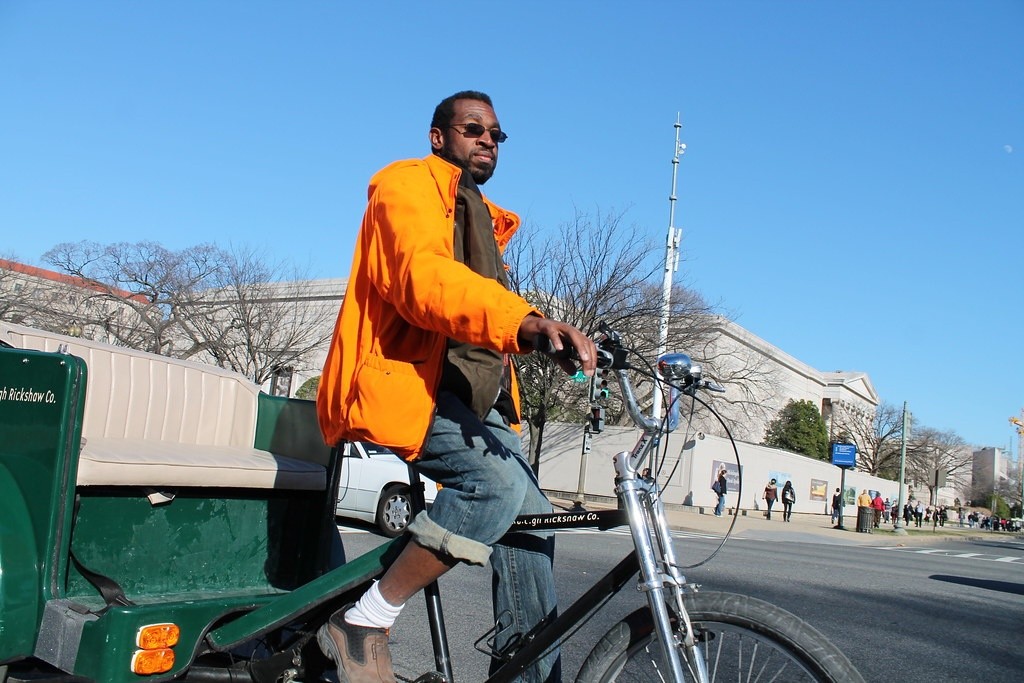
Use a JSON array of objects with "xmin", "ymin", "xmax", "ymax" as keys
[{"xmin": 856, "ymin": 506, "xmax": 876, "ymax": 532}]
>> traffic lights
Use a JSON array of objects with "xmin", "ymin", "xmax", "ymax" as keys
[
  {"xmin": 588, "ymin": 408, "xmax": 605, "ymax": 431},
  {"xmin": 587, "ymin": 367, "xmax": 611, "ymax": 405}
]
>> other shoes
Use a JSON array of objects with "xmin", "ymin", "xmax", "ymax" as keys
[
  {"xmin": 712, "ymin": 508, "xmax": 716, "ymax": 514},
  {"xmin": 716, "ymin": 515, "xmax": 723, "ymax": 517},
  {"xmin": 874, "ymin": 524, "xmax": 879, "ymax": 528}
]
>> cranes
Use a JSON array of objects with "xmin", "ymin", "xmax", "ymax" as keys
[{"xmin": 1009, "ymin": 406, "xmax": 1024, "ymax": 504}]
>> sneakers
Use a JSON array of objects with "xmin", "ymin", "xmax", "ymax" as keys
[{"xmin": 317, "ymin": 602, "xmax": 393, "ymax": 683}]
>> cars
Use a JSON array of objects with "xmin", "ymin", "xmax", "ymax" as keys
[{"xmin": 336, "ymin": 440, "xmax": 448, "ymax": 537}]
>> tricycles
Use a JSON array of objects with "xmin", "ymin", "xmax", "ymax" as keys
[{"xmin": 0, "ymin": 320, "xmax": 865, "ymax": 683}]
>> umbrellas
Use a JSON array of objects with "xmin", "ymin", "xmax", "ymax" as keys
[{"xmin": 968, "ymin": 515, "xmax": 978, "ymax": 522}]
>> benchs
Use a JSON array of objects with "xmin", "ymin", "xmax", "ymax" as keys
[{"xmin": 0, "ymin": 320, "xmax": 345, "ymax": 491}]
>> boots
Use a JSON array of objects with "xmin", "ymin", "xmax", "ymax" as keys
[
  {"xmin": 784, "ymin": 513, "xmax": 786, "ymax": 522},
  {"xmin": 788, "ymin": 512, "xmax": 791, "ymax": 521}
]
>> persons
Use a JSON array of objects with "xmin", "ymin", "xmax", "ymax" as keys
[
  {"xmin": 969, "ymin": 518, "xmax": 974, "ymax": 528},
  {"xmin": 959, "ymin": 507, "xmax": 965, "ymax": 527},
  {"xmin": 762, "ymin": 479, "xmax": 778, "ymax": 520},
  {"xmin": 870, "ymin": 492, "xmax": 885, "ymax": 528},
  {"xmin": 980, "ymin": 517, "xmax": 1021, "ymax": 532},
  {"xmin": 858, "ymin": 489, "xmax": 872, "ymax": 507},
  {"xmin": 891, "ymin": 499, "xmax": 898, "ymax": 524},
  {"xmin": 831, "ymin": 488, "xmax": 845, "ymax": 524},
  {"xmin": 903, "ymin": 500, "xmax": 948, "ymax": 527},
  {"xmin": 712, "ymin": 470, "xmax": 727, "ymax": 517},
  {"xmin": 318, "ymin": 90, "xmax": 597, "ymax": 683},
  {"xmin": 782, "ymin": 481, "xmax": 795, "ymax": 522},
  {"xmin": 883, "ymin": 498, "xmax": 891, "ymax": 523}
]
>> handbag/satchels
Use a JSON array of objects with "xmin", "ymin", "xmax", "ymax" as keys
[
  {"xmin": 785, "ymin": 489, "xmax": 795, "ymax": 502},
  {"xmin": 712, "ymin": 481, "xmax": 722, "ymax": 494}
]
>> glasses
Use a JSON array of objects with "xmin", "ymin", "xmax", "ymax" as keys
[{"xmin": 441, "ymin": 122, "xmax": 509, "ymax": 143}]
